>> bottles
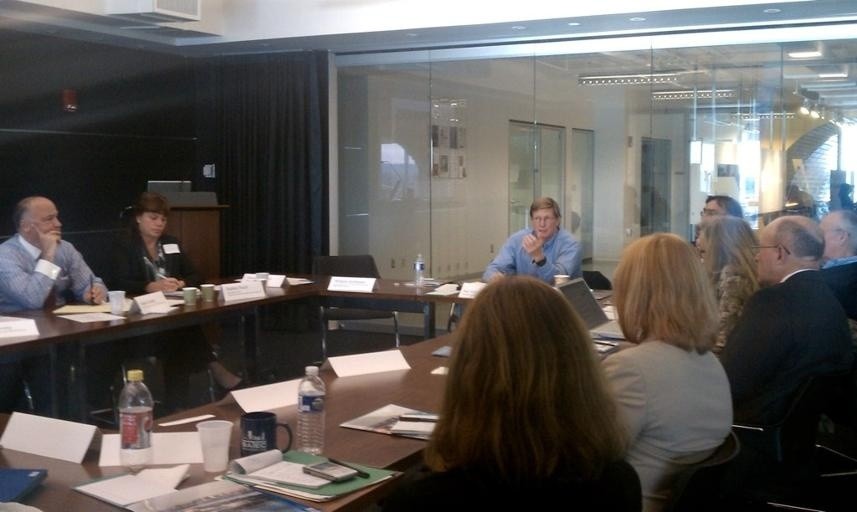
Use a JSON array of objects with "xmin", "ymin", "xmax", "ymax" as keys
[
  {"xmin": 299, "ymin": 367, "xmax": 327, "ymax": 457},
  {"xmin": 118, "ymin": 369, "xmax": 154, "ymax": 472},
  {"xmin": 413, "ymin": 252, "xmax": 425, "ymax": 291}
]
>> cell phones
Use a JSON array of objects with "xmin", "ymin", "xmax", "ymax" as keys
[
  {"xmin": 399, "ymin": 412, "xmax": 440, "ymax": 422},
  {"xmin": 302, "ymin": 462, "xmax": 359, "ymax": 482}
]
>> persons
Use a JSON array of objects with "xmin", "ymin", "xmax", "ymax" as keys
[
  {"xmin": 111, "ymin": 191, "xmax": 205, "ymax": 293},
  {"xmin": 598, "ymin": 233, "xmax": 732, "ymax": 511},
  {"xmin": 720, "ymin": 217, "xmax": 852, "ymax": 447},
  {"xmin": 818, "ymin": 211, "xmax": 857, "ymax": 323},
  {"xmin": 698, "ymin": 219, "xmax": 761, "ymax": 356},
  {"xmin": 371, "ymin": 274, "xmax": 645, "ymax": 512},
  {"xmin": 0, "ymin": 196, "xmax": 109, "ymax": 309},
  {"xmin": 701, "ymin": 196, "xmax": 741, "ymax": 220},
  {"xmin": 482, "ymin": 197, "xmax": 584, "ymax": 285}
]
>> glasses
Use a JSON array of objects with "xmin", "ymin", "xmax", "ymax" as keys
[
  {"xmin": 819, "ymin": 228, "xmax": 852, "ymax": 236},
  {"xmin": 751, "ymin": 242, "xmax": 791, "ymax": 256},
  {"xmin": 700, "ymin": 211, "xmax": 728, "ymax": 218}
]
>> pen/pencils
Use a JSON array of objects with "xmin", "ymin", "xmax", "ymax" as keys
[
  {"xmin": 593, "ymin": 340, "xmax": 615, "ymax": 346},
  {"xmin": 89, "ymin": 273, "xmax": 96, "ymax": 305},
  {"xmin": 156, "ymin": 273, "xmax": 166, "ymax": 279},
  {"xmin": 328, "ymin": 457, "xmax": 369, "ymax": 478},
  {"xmin": 399, "ymin": 416, "xmax": 438, "ymax": 421}
]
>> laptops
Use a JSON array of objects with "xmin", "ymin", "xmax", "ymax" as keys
[{"xmin": 555, "ymin": 277, "xmax": 624, "ymax": 339}]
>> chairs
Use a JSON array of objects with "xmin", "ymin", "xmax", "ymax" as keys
[
  {"xmin": 732, "ymin": 370, "xmax": 857, "ymax": 490},
  {"xmin": 666, "ymin": 432, "xmax": 740, "ymax": 512},
  {"xmin": 312, "ymin": 255, "xmax": 399, "ymax": 364}
]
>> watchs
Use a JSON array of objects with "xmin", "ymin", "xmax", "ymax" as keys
[{"xmin": 531, "ymin": 257, "xmax": 549, "ymax": 267}]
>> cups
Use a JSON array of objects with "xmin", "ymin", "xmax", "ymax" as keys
[
  {"xmin": 263, "ymin": 280, "xmax": 267, "ymax": 291},
  {"xmin": 183, "ymin": 287, "xmax": 199, "ymax": 305},
  {"xmin": 200, "ymin": 284, "xmax": 215, "ymax": 302},
  {"xmin": 554, "ymin": 275, "xmax": 569, "ymax": 285},
  {"xmin": 257, "ymin": 273, "xmax": 269, "ymax": 279},
  {"xmin": 239, "ymin": 410, "xmax": 294, "ymax": 458},
  {"xmin": 196, "ymin": 419, "xmax": 233, "ymax": 474},
  {"xmin": 108, "ymin": 290, "xmax": 127, "ymax": 314}
]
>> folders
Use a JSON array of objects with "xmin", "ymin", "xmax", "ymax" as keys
[{"xmin": 220, "ymin": 448, "xmax": 404, "ymax": 503}]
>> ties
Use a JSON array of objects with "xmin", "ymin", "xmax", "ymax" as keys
[{"xmin": 38, "ymin": 253, "xmax": 56, "ymax": 308}]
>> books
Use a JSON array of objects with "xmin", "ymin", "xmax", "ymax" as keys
[
  {"xmin": 216, "ymin": 446, "xmax": 403, "ymax": 501},
  {"xmin": 70, "ymin": 474, "xmax": 179, "ymax": 507},
  {"xmin": 57, "ymin": 312, "xmax": 128, "ymax": 324},
  {"xmin": 123, "ymin": 480, "xmax": 321, "ymax": 512},
  {"xmin": 339, "ymin": 403, "xmax": 443, "ymax": 440},
  {"xmin": 54, "ymin": 303, "xmax": 109, "ymax": 313},
  {"xmin": 0, "ymin": 468, "xmax": 50, "ymax": 503},
  {"xmin": 593, "ymin": 339, "xmax": 618, "ymax": 352},
  {"xmin": 139, "ymin": 464, "xmax": 190, "ymax": 488}
]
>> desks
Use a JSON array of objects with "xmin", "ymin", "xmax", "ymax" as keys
[{"xmin": 0, "ymin": 279, "xmax": 639, "ymax": 512}]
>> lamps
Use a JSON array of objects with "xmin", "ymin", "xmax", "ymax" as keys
[
  {"xmin": 818, "ymin": 64, "xmax": 848, "ymax": 79},
  {"xmin": 786, "ymin": 43, "xmax": 822, "ymax": 60},
  {"xmin": 577, "ymin": 74, "xmax": 680, "ymax": 87},
  {"xmin": 800, "ymin": 100, "xmax": 825, "ymax": 120},
  {"xmin": 731, "ymin": 112, "xmax": 796, "ymax": 120},
  {"xmin": 651, "ymin": 89, "xmax": 752, "ymax": 100}
]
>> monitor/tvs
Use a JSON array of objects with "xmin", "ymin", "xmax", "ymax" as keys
[{"xmin": 148, "ymin": 180, "xmax": 190, "ymax": 194}]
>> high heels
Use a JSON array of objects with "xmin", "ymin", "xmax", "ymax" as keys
[
  {"xmin": 216, "ymin": 370, "xmax": 251, "ymax": 390},
  {"xmin": 533, "ymin": 215, "xmax": 559, "ymax": 221}
]
